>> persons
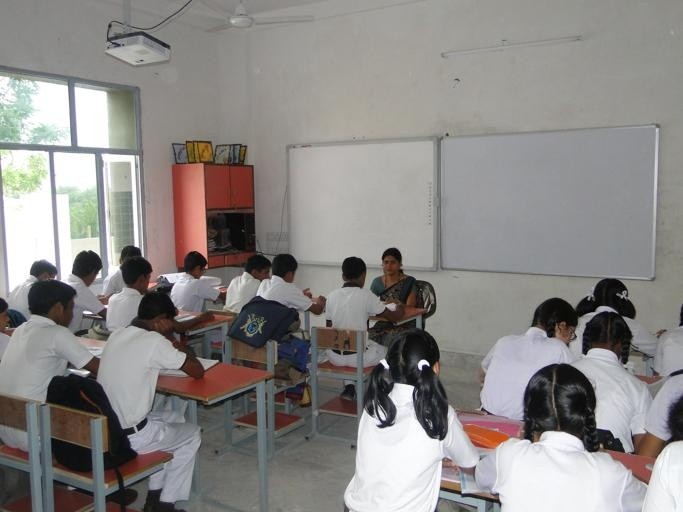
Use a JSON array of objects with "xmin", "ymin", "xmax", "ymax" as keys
[
  {"xmin": 477, "ymin": 278, "xmax": 683, "ymax": 512},
  {"xmin": 368, "ymin": 246, "xmax": 418, "ymax": 346},
  {"xmin": 325, "ymin": 256, "xmax": 392, "ymax": 403},
  {"xmin": 97, "ymin": 292, "xmax": 205, "ymax": 512},
  {"xmin": 0, "ymin": 279, "xmax": 100, "ymax": 453},
  {"xmin": 8, "ymin": 245, "xmax": 153, "ymax": 333},
  {"xmin": 0, "ymin": 298, "xmax": 16, "ymax": 352},
  {"xmin": 170, "ymin": 252, "xmax": 326, "ymax": 345},
  {"xmin": 343, "ymin": 327, "xmax": 479, "ymax": 511}
]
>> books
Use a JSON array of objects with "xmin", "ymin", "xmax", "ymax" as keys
[{"xmin": 458, "ymin": 467, "xmax": 489, "ymax": 495}]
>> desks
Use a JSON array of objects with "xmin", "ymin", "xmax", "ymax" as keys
[
  {"xmin": 293, "ymin": 295, "xmax": 429, "ymax": 342},
  {"xmin": 46, "ymin": 277, "xmax": 242, "ymax": 331},
  {"xmin": 17, "ymin": 333, "xmax": 277, "ymax": 512},
  {"xmin": 634, "ymin": 373, "xmax": 666, "ymax": 387},
  {"xmin": 427, "ymin": 450, "xmax": 683, "ymax": 512}
]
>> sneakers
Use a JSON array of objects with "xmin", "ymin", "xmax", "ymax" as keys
[
  {"xmin": 111, "ymin": 489, "xmax": 137, "ymax": 503},
  {"xmin": 341, "ymin": 384, "xmax": 355, "ymax": 401},
  {"xmin": 142, "ymin": 500, "xmax": 184, "ymax": 512}
]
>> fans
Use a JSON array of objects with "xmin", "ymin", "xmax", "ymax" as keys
[{"xmin": 191, "ymin": 0, "xmax": 315, "ymax": 34}]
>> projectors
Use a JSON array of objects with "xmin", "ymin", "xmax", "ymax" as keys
[{"xmin": 105, "ymin": 31, "xmax": 171, "ymax": 66}]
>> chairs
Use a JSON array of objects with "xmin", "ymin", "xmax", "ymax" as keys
[
  {"xmin": 369, "ymin": 280, "xmax": 436, "ymax": 328},
  {"xmin": 306, "ymin": 326, "xmax": 377, "ymax": 444},
  {"xmin": 223, "ymin": 331, "xmax": 309, "ymax": 440},
  {"xmin": 0, "ymin": 395, "xmax": 175, "ymax": 511}
]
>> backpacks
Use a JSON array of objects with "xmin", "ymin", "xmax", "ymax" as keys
[{"xmin": 45, "ymin": 374, "xmax": 136, "ymax": 472}]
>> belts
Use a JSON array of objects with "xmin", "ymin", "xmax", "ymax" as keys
[{"xmin": 122, "ymin": 418, "xmax": 147, "ymax": 435}]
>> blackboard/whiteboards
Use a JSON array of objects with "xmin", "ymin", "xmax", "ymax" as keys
[
  {"xmin": 286, "ymin": 136, "xmax": 440, "ymax": 272},
  {"xmin": 440, "ymin": 124, "xmax": 660, "ymax": 282}
]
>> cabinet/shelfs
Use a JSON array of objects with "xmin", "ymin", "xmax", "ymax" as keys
[{"xmin": 172, "ymin": 164, "xmax": 255, "ymax": 268}]
